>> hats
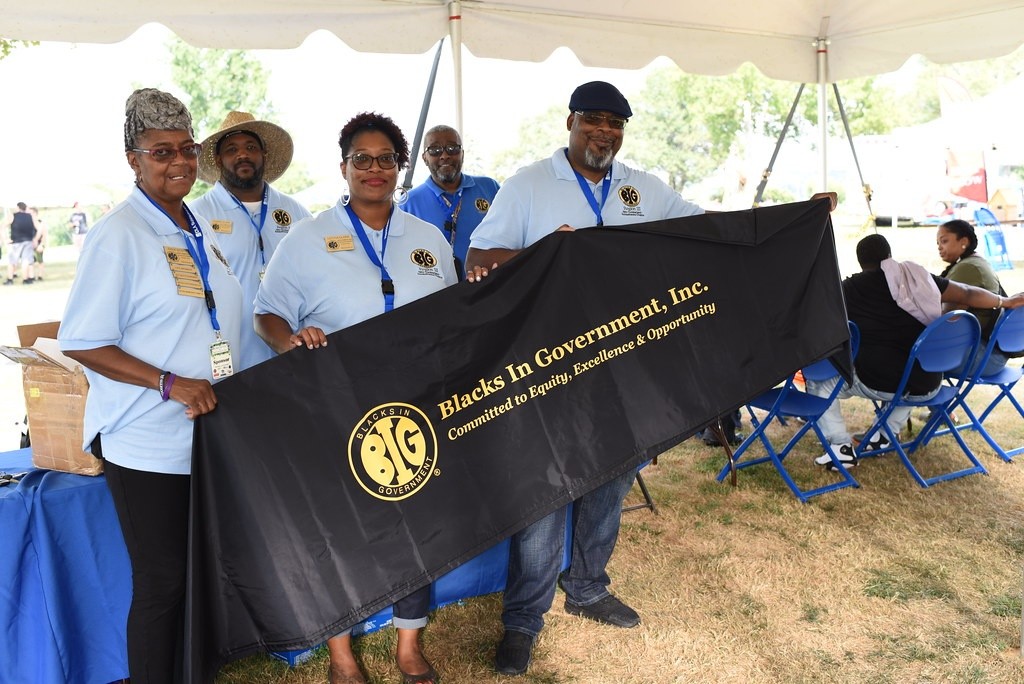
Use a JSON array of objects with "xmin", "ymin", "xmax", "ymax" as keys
[
  {"xmin": 569, "ymin": 81, "xmax": 633, "ymax": 120},
  {"xmin": 197, "ymin": 111, "xmax": 293, "ymax": 185}
]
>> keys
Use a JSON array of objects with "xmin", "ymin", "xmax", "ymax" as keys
[{"xmin": 0, "ymin": 470, "xmax": 28, "ymax": 487}]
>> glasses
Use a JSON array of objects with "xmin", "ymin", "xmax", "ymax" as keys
[
  {"xmin": 574, "ymin": 110, "xmax": 630, "ymax": 130},
  {"xmin": 424, "ymin": 144, "xmax": 462, "ymax": 157},
  {"xmin": 126, "ymin": 142, "xmax": 203, "ymax": 163},
  {"xmin": 344, "ymin": 152, "xmax": 401, "ymax": 170}
]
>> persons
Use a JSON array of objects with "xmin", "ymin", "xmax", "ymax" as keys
[
  {"xmin": 462, "ymin": 80, "xmax": 837, "ymax": 674},
  {"xmin": 3, "ymin": 202, "xmax": 47, "ymax": 286},
  {"xmin": 56, "ymin": 89, "xmax": 245, "ymax": 684},
  {"xmin": 186, "ymin": 110, "xmax": 314, "ymax": 370},
  {"xmin": 805, "ymin": 219, "xmax": 1024, "ymax": 470},
  {"xmin": 702, "ymin": 408, "xmax": 744, "ymax": 445},
  {"xmin": 67, "ymin": 200, "xmax": 87, "ymax": 254},
  {"xmin": 251, "ymin": 112, "xmax": 459, "ymax": 684},
  {"xmin": 397, "ymin": 125, "xmax": 500, "ymax": 279}
]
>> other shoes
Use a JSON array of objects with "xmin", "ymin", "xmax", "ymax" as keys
[
  {"xmin": 919, "ymin": 409, "xmax": 960, "ymax": 425},
  {"xmin": 23, "ymin": 279, "xmax": 31, "ymax": 284},
  {"xmin": 3, "ymin": 279, "xmax": 14, "ymax": 285}
]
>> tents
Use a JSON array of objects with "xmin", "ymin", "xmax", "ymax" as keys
[{"xmin": 0, "ymin": 0, "xmax": 1024, "ymax": 238}]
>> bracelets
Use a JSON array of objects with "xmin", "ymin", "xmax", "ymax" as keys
[
  {"xmin": 160, "ymin": 371, "xmax": 176, "ymax": 400},
  {"xmin": 993, "ymin": 295, "xmax": 1002, "ymax": 310}
]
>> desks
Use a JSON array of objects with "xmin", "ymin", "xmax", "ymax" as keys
[{"xmin": 0, "ymin": 434, "xmax": 578, "ymax": 683}]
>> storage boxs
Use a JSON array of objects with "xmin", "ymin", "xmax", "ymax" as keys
[{"xmin": 0, "ymin": 321, "xmax": 103, "ymax": 476}]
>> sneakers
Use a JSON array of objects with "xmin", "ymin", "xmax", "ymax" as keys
[
  {"xmin": 851, "ymin": 425, "xmax": 891, "ymax": 456},
  {"xmin": 396, "ymin": 650, "xmax": 439, "ymax": 684},
  {"xmin": 495, "ymin": 629, "xmax": 538, "ymax": 675},
  {"xmin": 814, "ymin": 442, "xmax": 860, "ymax": 471},
  {"xmin": 564, "ymin": 594, "xmax": 640, "ymax": 627}
]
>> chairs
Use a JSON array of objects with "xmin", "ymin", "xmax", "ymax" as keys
[
  {"xmin": 921, "ymin": 299, "xmax": 1024, "ymax": 465},
  {"xmin": 715, "ymin": 320, "xmax": 862, "ymax": 504},
  {"xmin": 976, "ymin": 210, "xmax": 1014, "ymax": 275},
  {"xmin": 852, "ymin": 311, "xmax": 985, "ymax": 494}
]
